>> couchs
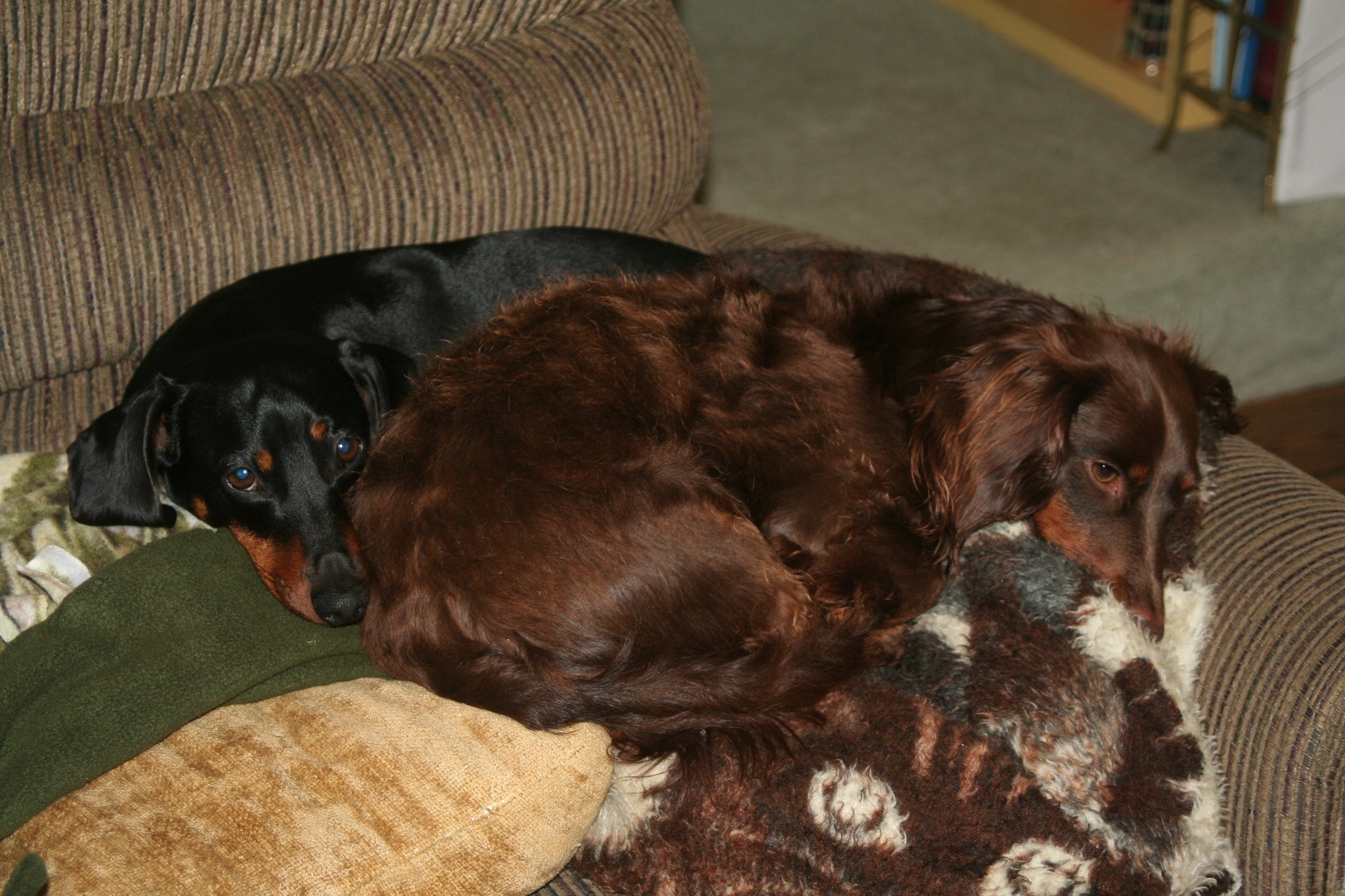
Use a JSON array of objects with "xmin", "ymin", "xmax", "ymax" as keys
[{"xmin": 1, "ymin": 0, "xmax": 1345, "ymax": 896}]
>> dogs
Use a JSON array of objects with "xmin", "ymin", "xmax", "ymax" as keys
[
  {"xmin": 68, "ymin": 225, "xmax": 716, "ymax": 628},
  {"xmin": 347, "ymin": 244, "xmax": 1253, "ymax": 766}
]
